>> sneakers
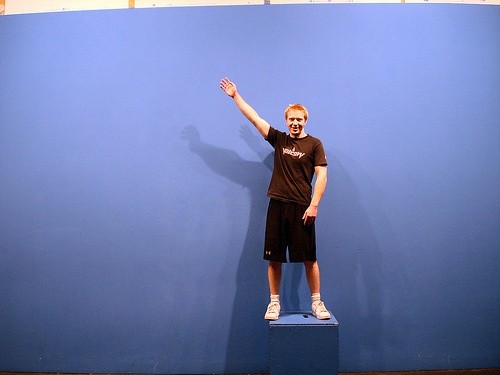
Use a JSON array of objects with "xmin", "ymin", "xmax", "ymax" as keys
[
  {"xmin": 264, "ymin": 302, "xmax": 280, "ymax": 320},
  {"xmin": 311, "ymin": 301, "xmax": 331, "ymax": 320}
]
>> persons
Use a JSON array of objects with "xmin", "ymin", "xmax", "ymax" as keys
[{"xmin": 220, "ymin": 78, "xmax": 331, "ymax": 321}]
